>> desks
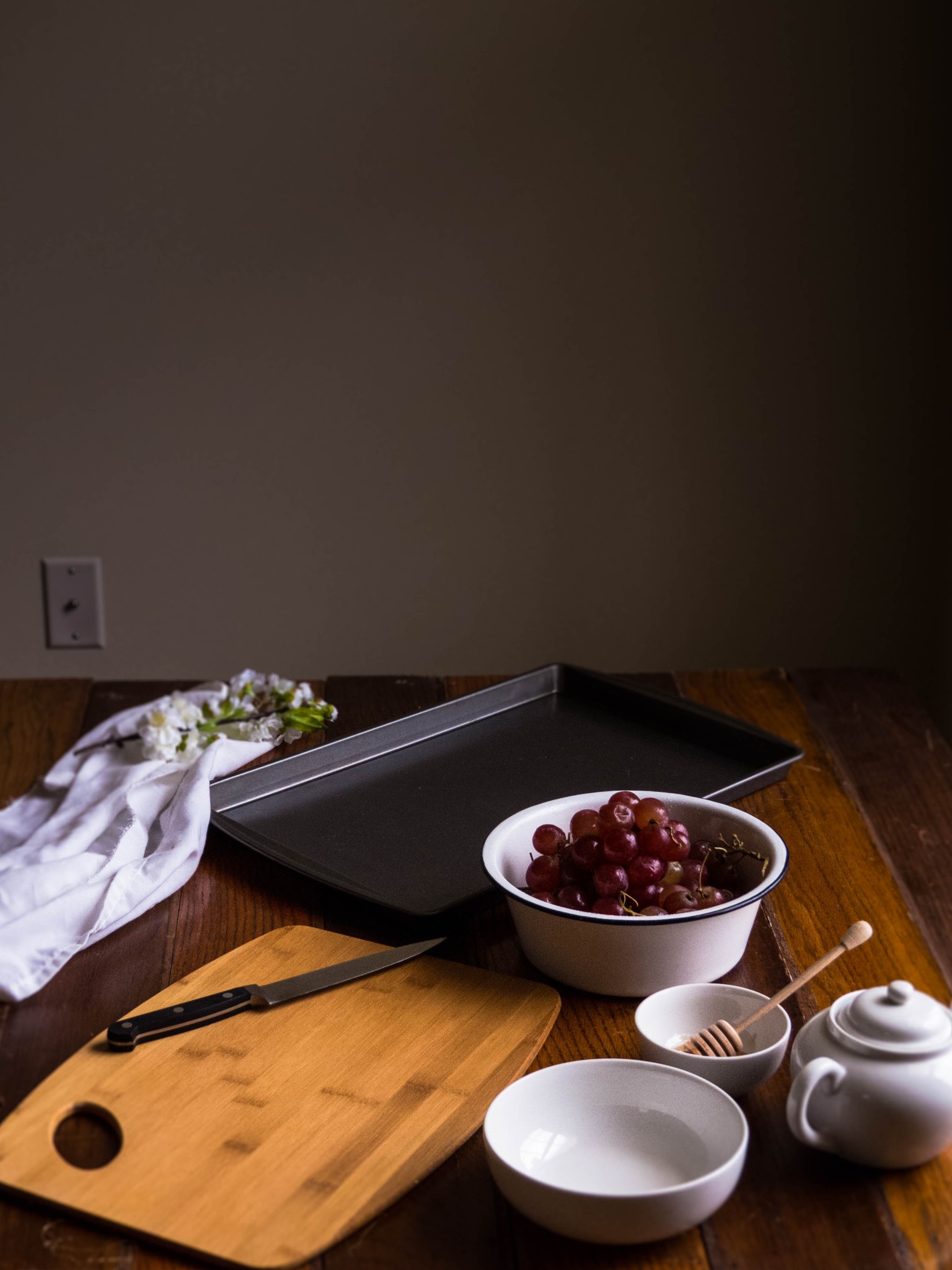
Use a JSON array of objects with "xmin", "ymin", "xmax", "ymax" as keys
[{"xmin": 0, "ymin": 662, "xmax": 952, "ymax": 1270}]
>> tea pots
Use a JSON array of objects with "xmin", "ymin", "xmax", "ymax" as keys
[{"xmin": 785, "ymin": 980, "xmax": 952, "ymax": 1170}]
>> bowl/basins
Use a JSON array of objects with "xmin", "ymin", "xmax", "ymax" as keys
[
  {"xmin": 480, "ymin": 1059, "xmax": 749, "ymax": 1245},
  {"xmin": 480, "ymin": 791, "xmax": 789, "ymax": 998},
  {"xmin": 633, "ymin": 984, "xmax": 793, "ymax": 1104}
]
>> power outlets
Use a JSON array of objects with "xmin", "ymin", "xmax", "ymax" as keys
[{"xmin": 43, "ymin": 558, "xmax": 106, "ymax": 649}]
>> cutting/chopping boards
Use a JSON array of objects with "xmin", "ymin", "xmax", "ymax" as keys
[{"xmin": 0, "ymin": 925, "xmax": 564, "ymax": 1270}]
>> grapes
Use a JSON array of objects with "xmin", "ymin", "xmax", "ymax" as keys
[{"xmin": 517, "ymin": 793, "xmax": 742, "ymax": 916}]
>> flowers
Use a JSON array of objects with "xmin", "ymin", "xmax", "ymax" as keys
[{"xmin": 70, "ymin": 674, "xmax": 338, "ymax": 767}]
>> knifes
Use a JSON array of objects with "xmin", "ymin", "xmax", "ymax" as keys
[{"xmin": 105, "ymin": 936, "xmax": 454, "ymax": 1051}]
